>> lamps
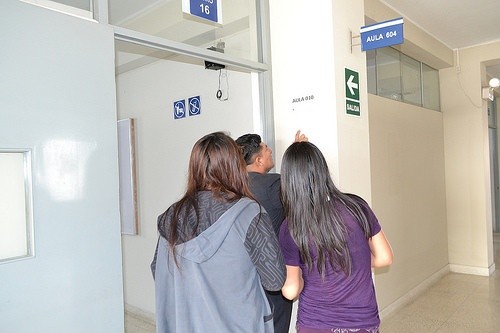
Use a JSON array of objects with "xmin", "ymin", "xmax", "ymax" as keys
[{"xmin": 482, "ymin": 78, "xmax": 500, "ymax": 102}]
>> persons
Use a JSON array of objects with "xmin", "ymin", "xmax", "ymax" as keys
[
  {"xmin": 234, "ymin": 133, "xmax": 308, "ymax": 333},
  {"xmin": 279, "ymin": 141, "xmax": 394, "ymax": 333},
  {"xmin": 149, "ymin": 131, "xmax": 286, "ymax": 333}
]
